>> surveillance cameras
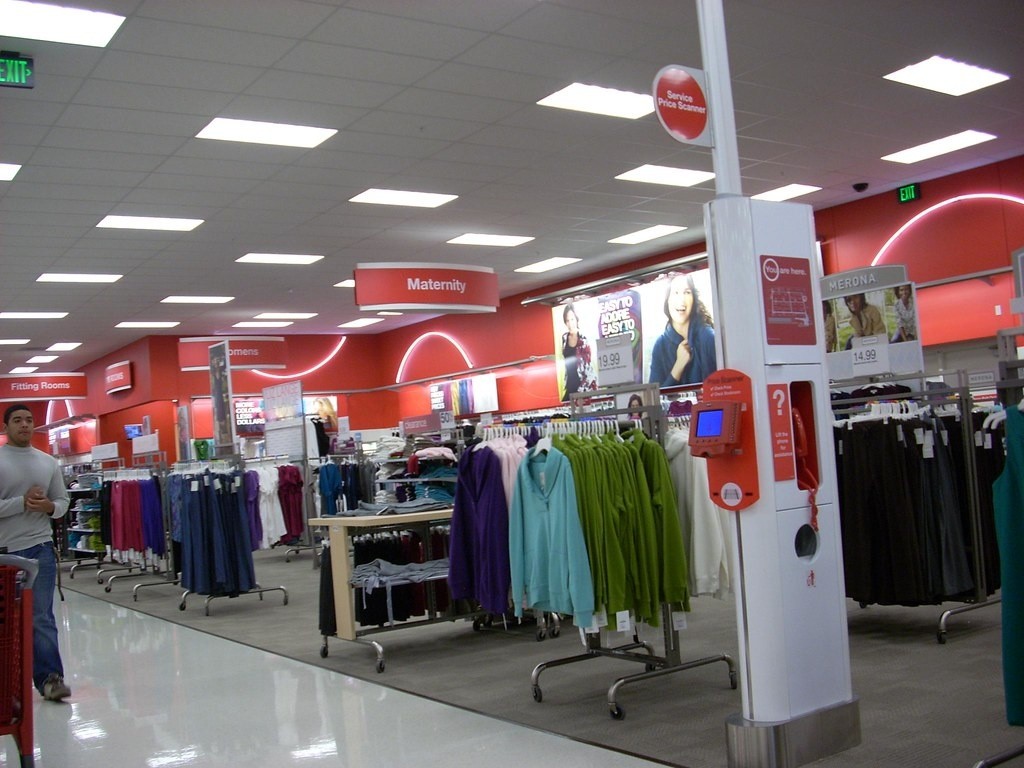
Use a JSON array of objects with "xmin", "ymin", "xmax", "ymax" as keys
[{"xmin": 853, "ymin": 183, "xmax": 868, "ymax": 192}]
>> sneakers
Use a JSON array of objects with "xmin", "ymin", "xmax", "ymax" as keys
[{"xmin": 43, "ymin": 672, "xmax": 71, "ymax": 701}]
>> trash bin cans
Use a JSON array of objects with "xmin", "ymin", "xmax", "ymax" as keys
[{"xmin": 776, "ymin": 503, "xmax": 857, "ymax": 716}]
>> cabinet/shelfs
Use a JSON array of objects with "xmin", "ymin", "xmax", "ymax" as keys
[
  {"xmin": 369, "ymin": 454, "xmax": 460, "ymax": 484},
  {"xmin": 308, "ymin": 507, "xmax": 514, "ymax": 641}
]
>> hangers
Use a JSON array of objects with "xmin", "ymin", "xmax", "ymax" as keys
[
  {"xmin": 319, "ymin": 456, "xmax": 374, "ymax": 464},
  {"xmin": 100, "ymin": 453, "xmax": 297, "ymax": 484},
  {"xmin": 833, "ymin": 399, "xmax": 1024, "ymax": 430},
  {"xmin": 468, "ymin": 412, "xmax": 695, "ymax": 460}
]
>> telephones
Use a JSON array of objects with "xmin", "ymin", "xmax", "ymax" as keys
[{"xmin": 791, "ymin": 405, "xmax": 808, "ymax": 462}]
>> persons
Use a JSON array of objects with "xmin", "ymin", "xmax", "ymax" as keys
[
  {"xmin": 649, "ymin": 275, "xmax": 717, "ymax": 388},
  {"xmin": 822, "ymin": 300, "xmax": 836, "ymax": 353},
  {"xmin": 560, "ymin": 304, "xmax": 598, "ymax": 402},
  {"xmin": 843, "ymin": 294, "xmax": 885, "ymax": 348},
  {"xmin": 0, "ymin": 406, "xmax": 70, "ymax": 699},
  {"xmin": 890, "ymin": 284, "xmax": 915, "ymax": 343},
  {"xmin": 627, "ymin": 394, "xmax": 642, "ymax": 419},
  {"xmin": 313, "ymin": 398, "xmax": 337, "ymax": 428}
]
